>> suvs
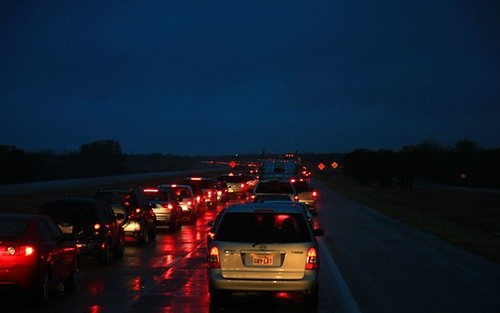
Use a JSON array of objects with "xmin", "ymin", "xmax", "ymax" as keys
[{"xmin": 92, "ymin": 187, "xmax": 158, "ymax": 246}]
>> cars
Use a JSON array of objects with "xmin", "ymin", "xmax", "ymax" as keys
[
  {"xmin": 178, "ymin": 157, "xmax": 325, "ymax": 251},
  {"xmin": 158, "ymin": 184, "xmax": 198, "ymax": 224},
  {"xmin": 141, "ymin": 187, "xmax": 185, "ymax": 233},
  {"xmin": 0, "ymin": 214, "xmax": 83, "ymax": 304},
  {"xmin": 35, "ymin": 196, "xmax": 126, "ymax": 265},
  {"xmin": 208, "ymin": 203, "xmax": 327, "ymax": 309}
]
